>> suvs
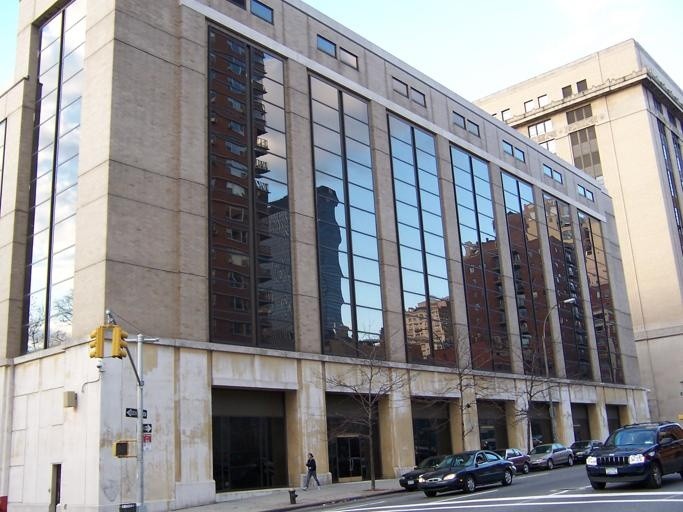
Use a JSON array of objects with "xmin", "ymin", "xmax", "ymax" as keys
[{"xmin": 585, "ymin": 421, "xmax": 683, "ymax": 488}]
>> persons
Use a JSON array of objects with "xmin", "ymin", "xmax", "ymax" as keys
[{"xmin": 300, "ymin": 452, "xmax": 322, "ymax": 492}]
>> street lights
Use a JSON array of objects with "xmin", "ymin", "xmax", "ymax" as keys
[{"xmin": 543, "ymin": 298, "xmax": 576, "ymax": 442}]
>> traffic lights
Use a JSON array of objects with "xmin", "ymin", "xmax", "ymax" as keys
[
  {"xmin": 90, "ymin": 325, "xmax": 105, "ymax": 358},
  {"xmin": 111, "ymin": 327, "xmax": 128, "ymax": 358}
]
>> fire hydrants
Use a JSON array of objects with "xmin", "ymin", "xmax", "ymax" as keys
[{"xmin": 288, "ymin": 488, "xmax": 297, "ymax": 504}]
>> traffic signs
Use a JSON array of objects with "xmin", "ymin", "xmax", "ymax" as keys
[
  {"xmin": 136, "ymin": 424, "xmax": 152, "ymax": 434},
  {"xmin": 125, "ymin": 407, "xmax": 147, "ymax": 418}
]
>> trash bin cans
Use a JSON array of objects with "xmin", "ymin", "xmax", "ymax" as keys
[
  {"xmin": 0, "ymin": 495, "xmax": 7, "ymax": 512},
  {"xmin": 118, "ymin": 502, "xmax": 137, "ymax": 511}
]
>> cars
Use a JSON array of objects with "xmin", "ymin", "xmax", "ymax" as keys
[
  {"xmin": 533, "ymin": 439, "xmax": 542, "ymax": 446},
  {"xmin": 527, "ymin": 442, "xmax": 574, "ymax": 469},
  {"xmin": 569, "ymin": 440, "xmax": 603, "ymax": 463},
  {"xmin": 400, "ymin": 448, "xmax": 529, "ymax": 496}
]
